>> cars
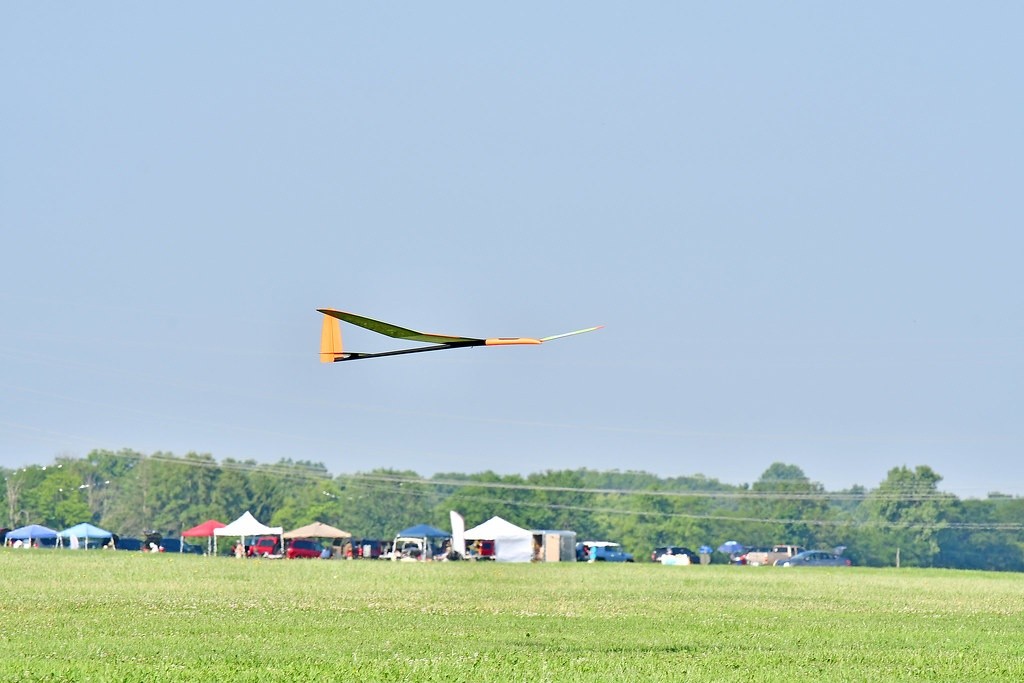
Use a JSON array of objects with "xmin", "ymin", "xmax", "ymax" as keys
[
  {"xmin": 729, "ymin": 546, "xmax": 773, "ymax": 565},
  {"xmin": 651, "ymin": 547, "xmax": 700, "ymax": 564},
  {"xmin": 575, "ymin": 541, "xmax": 633, "ymax": 562},
  {"xmin": 340, "ymin": 539, "xmax": 384, "ymax": 557},
  {"xmin": 249, "ymin": 536, "xmax": 279, "ymax": 556},
  {"xmin": 773, "ymin": 546, "xmax": 851, "ymax": 566},
  {"xmin": 276, "ymin": 538, "xmax": 326, "ymax": 559},
  {"xmin": 32, "ymin": 538, "xmax": 70, "ymax": 547},
  {"xmin": 79, "ymin": 541, "xmax": 100, "ymax": 549},
  {"xmin": 159, "ymin": 538, "xmax": 203, "ymax": 554},
  {"xmin": 115, "ymin": 539, "xmax": 145, "ymax": 550}
]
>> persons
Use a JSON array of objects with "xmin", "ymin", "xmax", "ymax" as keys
[{"xmin": 236, "ymin": 540, "xmax": 244, "ymax": 558}]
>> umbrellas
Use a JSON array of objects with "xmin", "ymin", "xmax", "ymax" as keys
[{"xmin": 718, "ymin": 541, "xmax": 745, "ymax": 565}]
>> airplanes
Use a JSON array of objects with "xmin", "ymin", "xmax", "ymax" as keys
[{"xmin": 316, "ymin": 308, "xmax": 606, "ymax": 363}]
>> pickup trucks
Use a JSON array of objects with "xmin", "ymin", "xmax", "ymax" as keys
[{"xmin": 746, "ymin": 544, "xmax": 806, "ymax": 566}]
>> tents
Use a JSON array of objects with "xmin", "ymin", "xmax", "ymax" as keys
[
  {"xmin": 392, "ymin": 525, "xmax": 453, "ymax": 562},
  {"xmin": 463, "ymin": 515, "xmax": 534, "ymax": 563},
  {"xmin": 213, "ymin": 511, "xmax": 284, "ymax": 558},
  {"xmin": 180, "ymin": 519, "xmax": 227, "ymax": 555},
  {"xmin": 283, "ymin": 521, "xmax": 352, "ymax": 559},
  {"xmin": 4, "ymin": 525, "xmax": 63, "ymax": 548},
  {"xmin": 55, "ymin": 523, "xmax": 116, "ymax": 552}
]
